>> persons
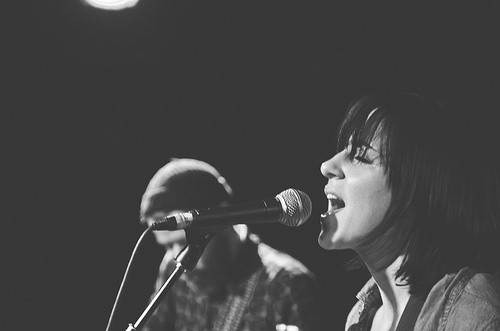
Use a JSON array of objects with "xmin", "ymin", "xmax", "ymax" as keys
[
  {"xmin": 141, "ymin": 158, "xmax": 328, "ymax": 331},
  {"xmin": 315, "ymin": 80, "xmax": 500, "ymax": 330}
]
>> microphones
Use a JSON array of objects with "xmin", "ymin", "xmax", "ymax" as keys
[{"xmin": 153, "ymin": 188, "xmax": 313, "ymax": 231}]
